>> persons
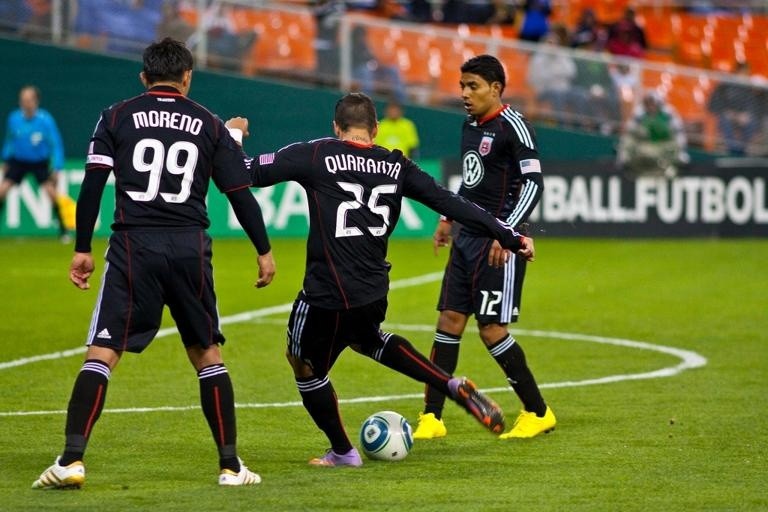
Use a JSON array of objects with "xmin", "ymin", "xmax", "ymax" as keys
[
  {"xmin": 0, "ymin": 84, "xmax": 72, "ymax": 245},
  {"xmin": 412, "ymin": 54, "xmax": 557, "ymax": 440},
  {"xmin": 29, "ymin": 37, "xmax": 264, "ymax": 491},
  {"xmin": 1, "ymin": 1, "xmax": 768, "ymax": 177},
  {"xmin": 222, "ymin": 91, "xmax": 535, "ymax": 467}
]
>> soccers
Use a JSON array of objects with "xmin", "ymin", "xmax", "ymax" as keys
[{"xmin": 360, "ymin": 411, "xmax": 414, "ymax": 462}]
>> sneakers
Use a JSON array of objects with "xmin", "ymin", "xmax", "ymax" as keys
[
  {"xmin": 309, "ymin": 447, "xmax": 363, "ymax": 466},
  {"xmin": 62, "ymin": 235, "xmax": 72, "ymax": 244},
  {"xmin": 218, "ymin": 456, "xmax": 261, "ymax": 486},
  {"xmin": 31, "ymin": 455, "xmax": 85, "ymax": 489},
  {"xmin": 412, "ymin": 411, "xmax": 447, "ymax": 439},
  {"xmin": 498, "ymin": 405, "xmax": 556, "ymax": 438},
  {"xmin": 448, "ymin": 377, "xmax": 505, "ymax": 433}
]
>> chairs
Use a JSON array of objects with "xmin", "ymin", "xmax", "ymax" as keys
[{"xmin": 177, "ymin": 0, "xmax": 766, "ymax": 157}]
